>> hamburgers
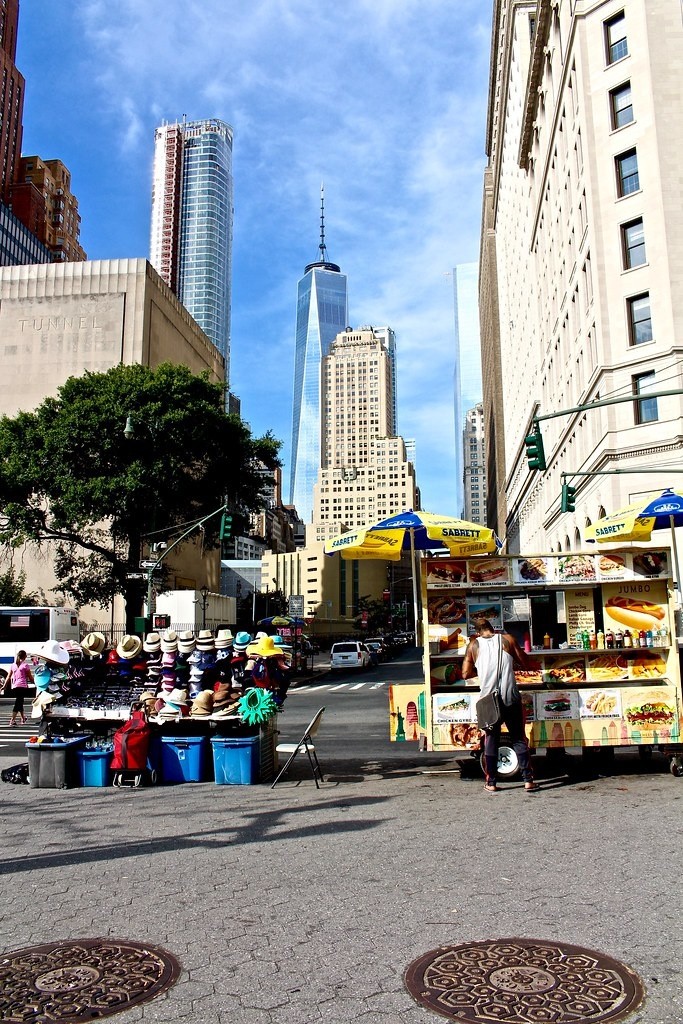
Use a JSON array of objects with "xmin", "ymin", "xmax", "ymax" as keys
[
  {"xmin": 469, "ymin": 561, "xmax": 506, "ymax": 583},
  {"xmin": 547, "ymin": 656, "xmax": 585, "ymax": 683},
  {"xmin": 430, "ymin": 563, "xmax": 462, "ymax": 583},
  {"xmin": 597, "ymin": 555, "xmax": 626, "ymax": 575},
  {"xmin": 519, "ymin": 692, "xmax": 534, "ymax": 717},
  {"xmin": 625, "ymin": 691, "xmax": 677, "ymax": 731},
  {"xmin": 543, "ymin": 693, "xmax": 571, "ymax": 716}
]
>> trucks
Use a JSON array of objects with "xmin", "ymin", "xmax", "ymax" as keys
[{"xmin": 154, "ymin": 590, "xmax": 236, "ymax": 636}]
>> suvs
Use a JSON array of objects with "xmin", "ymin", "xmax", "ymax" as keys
[{"xmin": 329, "ymin": 641, "xmax": 372, "ymax": 669}]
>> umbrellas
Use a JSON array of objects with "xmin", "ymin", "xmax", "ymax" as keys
[
  {"xmin": 324, "ymin": 509, "xmax": 501, "ymax": 647},
  {"xmin": 584, "ymin": 489, "xmax": 683, "ymax": 604},
  {"xmin": 257, "ymin": 615, "xmax": 307, "ymax": 627}
]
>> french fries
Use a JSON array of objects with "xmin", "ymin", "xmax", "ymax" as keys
[{"xmin": 590, "ymin": 655, "xmax": 625, "ymax": 678}]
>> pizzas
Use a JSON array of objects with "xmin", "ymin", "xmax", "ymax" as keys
[{"xmin": 448, "ymin": 724, "xmax": 481, "ymax": 748}]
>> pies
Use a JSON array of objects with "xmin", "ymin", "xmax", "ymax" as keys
[
  {"xmin": 431, "ymin": 663, "xmax": 462, "ymax": 685},
  {"xmin": 439, "ymin": 699, "xmax": 469, "ymax": 716},
  {"xmin": 470, "ymin": 606, "xmax": 500, "ymax": 622}
]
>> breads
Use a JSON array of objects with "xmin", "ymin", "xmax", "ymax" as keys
[{"xmin": 428, "ymin": 596, "xmax": 467, "ymax": 624}]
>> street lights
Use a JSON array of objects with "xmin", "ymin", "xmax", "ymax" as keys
[{"xmin": 199, "ymin": 585, "xmax": 210, "ymax": 629}]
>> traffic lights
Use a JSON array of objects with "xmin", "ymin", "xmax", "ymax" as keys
[
  {"xmin": 525, "ymin": 432, "xmax": 547, "ymax": 471},
  {"xmin": 126, "ymin": 573, "xmax": 149, "ymax": 580},
  {"xmin": 139, "ymin": 560, "xmax": 163, "ymax": 569},
  {"xmin": 382, "ymin": 591, "xmax": 390, "ymax": 609},
  {"xmin": 219, "ymin": 513, "xmax": 233, "ymax": 540},
  {"xmin": 561, "ymin": 484, "xmax": 576, "ymax": 513},
  {"xmin": 155, "ymin": 616, "xmax": 170, "ymax": 628}
]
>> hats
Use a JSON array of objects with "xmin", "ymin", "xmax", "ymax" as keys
[{"xmin": 30, "ymin": 630, "xmax": 293, "ymax": 727}]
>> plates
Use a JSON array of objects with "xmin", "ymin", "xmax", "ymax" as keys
[{"xmin": 425, "ymin": 549, "xmax": 668, "ymax": 720}]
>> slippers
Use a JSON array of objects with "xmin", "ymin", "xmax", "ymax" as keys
[
  {"xmin": 484, "ymin": 784, "xmax": 496, "ymax": 792},
  {"xmin": 524, "ymin": 783, "xmax": 539, "ymax": 790}
]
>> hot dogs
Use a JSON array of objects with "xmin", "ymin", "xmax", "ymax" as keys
[
  {"xmin": 586, "ymin": 692, "xmax": 605, "ymax": 712},
  {"xmin": 605, "ymin": 596, "xmax": 666, "ymax": 631}
]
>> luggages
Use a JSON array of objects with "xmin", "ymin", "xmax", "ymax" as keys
[{"xmin": 110, "ymin": 701, "xmax": 157, "ymax": 788}]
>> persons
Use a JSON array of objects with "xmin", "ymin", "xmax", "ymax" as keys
[
  {"xmin": 0, "ymin": 650, "xmax": 34, "ymax": 726},
  {"xmin": 462, "ymin": 619, "xmax": 540, "ymax": 792}
]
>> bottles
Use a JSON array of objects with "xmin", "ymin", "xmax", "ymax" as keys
[
  {"xmin": 660, "ymin": 623, "xmax": 668, "ymax": 647},
  {"xmin": 543, "ymin": 632, "xmax": 550, "ymax": 650},
  {"xmin": 596, "ymin": 629, "xmax": 605, "ymax": 649},
  {"xmin": 652, "ymin": 624, "xmax": 660, "ymax": 647},
  {"xmin": 583, "ymin": 630, "xmax": 591, "ymax": 649},
  {"xmin": 605, "ymin": 628, "xmax": 614, "ymax": 649},
  {"xmin": 590, "ymin": 630, "xmax": 596, "ymax": 649},
  {"xmin": 576, "ymin": 627, "xmax": 582, "ymax": 650},
  {"xmin": 614, "ymin": 628, "xmax": 623, "ymax": 649},
  {"xmin": 639, "ymin": 629, "xmax": 647, "ymax": 648},
  {"xmin": 632, "ymin": 629, "xmax": 640, "ymax": 648},
  {"xmin": 645, "ymin": 629, "xmax": 653, "ymax": 648},
  {"xmin": 623, "ymin": 629, "xmax": 633, "ymax": 648},
  {"xmin": 548, "ymin": 718, "xmax": 680, "ymax": 747}
]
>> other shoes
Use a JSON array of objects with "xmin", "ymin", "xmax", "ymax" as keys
[
  {"xmin": 22, "ymin": 718, "xmax": 27, "ymax": 724},
  {"xmin": 476, "ymin": 692, "xmax": 502, "ymax": 730},
  {"xmin": 10, "ymin": 721, "xmax": 17, "ymax": 726}
]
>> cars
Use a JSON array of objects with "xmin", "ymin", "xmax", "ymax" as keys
[{"xmin": 364, "ymin": 632, "xmax": 415, "ymax": 665}]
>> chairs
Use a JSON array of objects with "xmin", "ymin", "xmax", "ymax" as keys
[{"xmin": 271, "ymin": 706, "xmax": 326, "ymax": 789}]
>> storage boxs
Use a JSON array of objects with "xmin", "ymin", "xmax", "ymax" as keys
[
  {"xmin": 76, "ymin": 747, "xmax": 114, "ymax": 788},
  {"xmin": 160, "ymin": 730, "xmax": 260, "ymax": 785},
  {"xmin": 25, "ymin": 732, "xmax": 92, "ymax": 789}
]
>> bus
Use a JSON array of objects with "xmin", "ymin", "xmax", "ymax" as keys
[{"xmin": 0, "ymin": 606, "xmax": 80, "ymax": 698}]
